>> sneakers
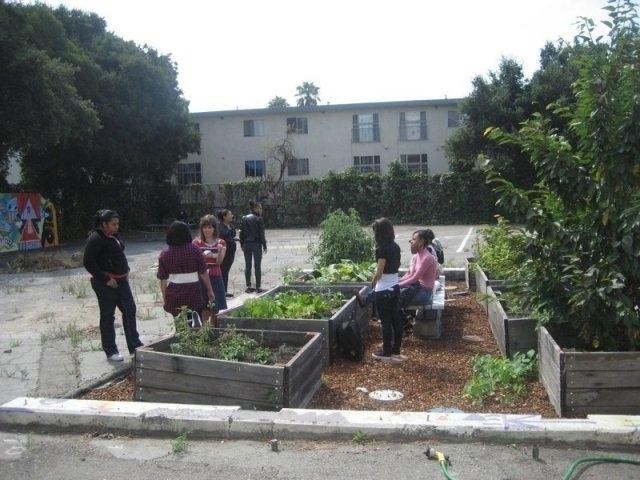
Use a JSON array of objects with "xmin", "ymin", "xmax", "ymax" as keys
[
  {"xmin": 226, "ymin": 293, "xmax": 233, "ymax": 297},
  {"xmin": 373, "ymin": 350, "xmax": 399, "ymax": 360},
  {"xmin": 192, "ymin": 310, "xmax": 202, "ymax": 328},
  {"xmin": 107, "ymin": 354, "xmax": 123, "ymax": 361},
  {"xmin": 245, "ymin": 287, "xmax": 263, "ymax": 293}
]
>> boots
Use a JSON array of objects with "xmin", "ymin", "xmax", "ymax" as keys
[{"xmin": 354, "ymin": 289, "xmax": 371, "ymax": 306}]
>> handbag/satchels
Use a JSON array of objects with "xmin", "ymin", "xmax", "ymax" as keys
[{"xmin": 336, "ymin": 321, "xmax": 361, "ymax": 362}]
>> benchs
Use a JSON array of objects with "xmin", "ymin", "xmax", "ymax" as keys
[{"xmin": 399, "ymin": 274, "xmax": 447, "ymax": 340}]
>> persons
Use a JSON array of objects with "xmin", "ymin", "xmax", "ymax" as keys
[
  {"xmin": 351, "ymin": 228, "xmax": 436, "ymax": 338},
  {"xmin": 369, "ymin": 217, "xmax": 404, "ymax": 361},
  {"xmin": 217, "ymin": 206, "xmax": 238, "ymax": 298},
  {"xmin": 82, "ymin": 208, "xmax": 144, "ymax": 363},
  {"xmin": 192, "ymin": 212, "xmax": 229, "ymax": 324},
  {"xmin": 239, "ymin": 199, "xmax": 268, "ymax": 293},
  {"xmin": 427, "ymin": 229, "xmax": 445, "ymax": 266},
  {"xmin": 156, "ymin": 219, "xmax": 216, "ymax": 335}
]
